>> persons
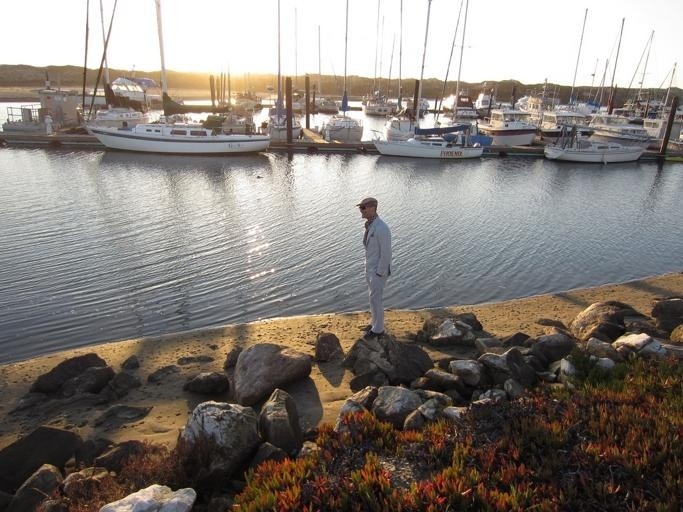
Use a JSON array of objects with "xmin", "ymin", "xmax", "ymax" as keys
[
  {"xmin": 44, "ymin": 114, "xmax": 53, "ymax": 135},
  {"xmin": 355, "ymin": 197, "xmax": 392, "ymax": 336}
]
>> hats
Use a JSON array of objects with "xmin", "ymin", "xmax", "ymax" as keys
[{"xmin": 356, "ymin": 198, "xmax": 377, "ymax": 206}]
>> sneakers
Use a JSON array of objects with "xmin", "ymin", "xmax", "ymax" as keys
[{"xmin": 361, "ymin": 325, "xmax": 384, "ymax": 339}]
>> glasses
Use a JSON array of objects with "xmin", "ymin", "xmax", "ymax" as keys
[{"xmin": 359, "ymin": 206, "xmax": 366, "ymax": 209}]
